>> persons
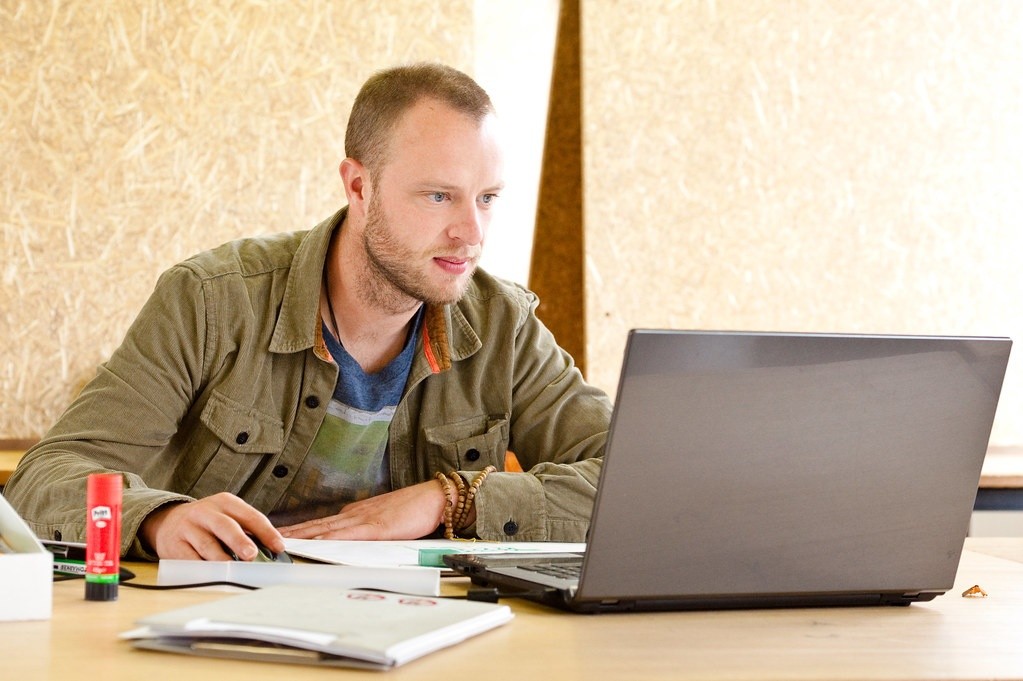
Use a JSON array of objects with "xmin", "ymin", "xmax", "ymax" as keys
[{"xmin": 4, "ymin": 63, "xmax": 612, "ymax": 561}]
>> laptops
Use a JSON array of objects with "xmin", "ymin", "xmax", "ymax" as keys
[{"xmin": 442, "ymin": 327, "xmax": 1014, "ymax": 615}]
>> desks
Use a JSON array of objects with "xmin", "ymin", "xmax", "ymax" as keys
[{"xmin": 0, "ymin": 442, "xmax": 1023, "ymax": 681}]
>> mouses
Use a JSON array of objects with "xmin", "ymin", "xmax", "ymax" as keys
[{"xmin": 217, "ymin": 532, "xmax": 294, "ymax": 564}]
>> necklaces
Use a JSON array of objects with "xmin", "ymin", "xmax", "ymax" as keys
[{"xmin": 324, "ymin": 271, "xmax": 346, "ymax": 349}]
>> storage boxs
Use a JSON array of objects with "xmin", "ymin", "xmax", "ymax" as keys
[{"xmin": 0, "ymin": 493, "xmax": 54, "ymax": 625}]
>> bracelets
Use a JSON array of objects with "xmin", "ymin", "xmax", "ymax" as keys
[{"xmin": 433, "ymin": 465, "xmax": 496, "ymax": 540}]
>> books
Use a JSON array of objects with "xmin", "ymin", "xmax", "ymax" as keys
[{"xmin": 117, "ymin": 583, "xmax": 512, "ymax": 671}]
